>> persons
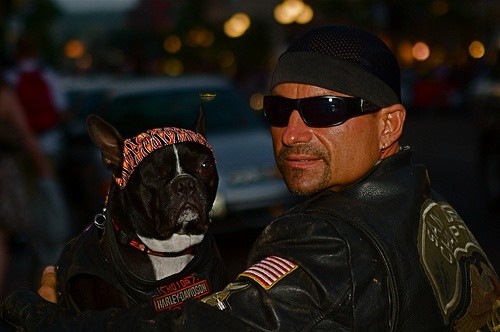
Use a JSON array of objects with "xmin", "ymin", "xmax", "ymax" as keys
[
  {"xmin": 0, "ymin": 25, "xmax": 500, "ymax": 332},
  {"xmin": 0, "ymin": 30, "xmax": 73, "ymax": 251}
]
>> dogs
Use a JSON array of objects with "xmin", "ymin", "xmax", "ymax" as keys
[{"xmin": 55, "ymin": 104, "xmax": 238, "ymax": 309}]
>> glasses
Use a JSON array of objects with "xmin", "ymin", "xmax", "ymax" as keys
[{"xmin": 263, "ymin": 95, "xmax": 382, "ymax": 129}]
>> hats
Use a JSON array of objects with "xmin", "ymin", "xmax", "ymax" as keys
[{"xmin": 272, "ymin": 24, "xmax": 402, "ymax": 109}]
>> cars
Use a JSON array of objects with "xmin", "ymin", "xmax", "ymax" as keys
[{"xmin": 43, "ymin": 72, "xmax": 305, "ymax": 256}]
